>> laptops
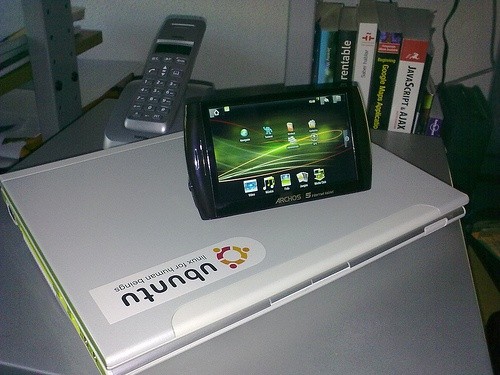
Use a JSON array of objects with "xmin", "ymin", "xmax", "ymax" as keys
[{"xmin": 1, "ymin": 129, "xmax": 472, "ymax": 375}]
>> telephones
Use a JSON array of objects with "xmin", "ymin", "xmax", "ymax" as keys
[{"xmin": 103, "ymin": 13, "xmax": 213, "ymax": 152}]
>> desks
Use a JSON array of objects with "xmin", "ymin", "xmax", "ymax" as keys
[{"xmin": 0, "ymin": 96, "xmax": 498, "ymax": 375}]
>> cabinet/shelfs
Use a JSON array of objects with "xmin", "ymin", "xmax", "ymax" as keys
[{"xmin": 0, "ymin": 0, "xmax": 134, "ymax": 176}]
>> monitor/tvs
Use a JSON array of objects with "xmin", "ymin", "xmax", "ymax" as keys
[{"xmin": 184, "ymin": 83, "xmax": 373, "ymax": 221}]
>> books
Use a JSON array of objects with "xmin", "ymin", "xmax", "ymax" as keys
[{"xmin": 312, "ymin": 1, "xmax": 445, "ymax": 137}]
